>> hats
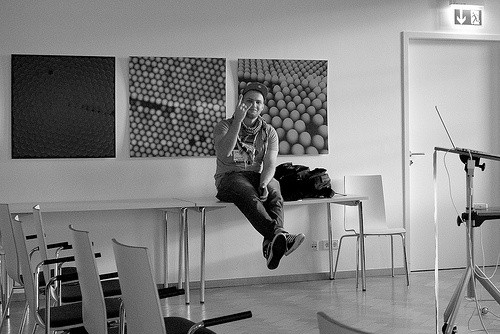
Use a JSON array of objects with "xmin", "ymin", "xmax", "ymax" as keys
[{"xmin": 242, "ymin": 83, "xmax": 268, "ymax": 101}]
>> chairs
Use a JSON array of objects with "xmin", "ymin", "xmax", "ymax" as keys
[
  {"xmin": 0, "ymin": 202, "xmax": 252, "ymax": 334},
  {"xmin": 333, "ymin": 175, "xmax": 410, "ymax": 292}
]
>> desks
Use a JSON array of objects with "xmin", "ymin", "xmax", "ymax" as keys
[
  {"xmin": 9, "ymin": 198, "xmax": 210, "ymax": 304},
  {"xmin": 179, "ymin": 192, "xmax": 370, "ymax": 308}
]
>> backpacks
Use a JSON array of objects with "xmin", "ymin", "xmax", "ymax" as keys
[{"xmin": 274, "ymin": 162, "xmax": 335, "ymax": 201}]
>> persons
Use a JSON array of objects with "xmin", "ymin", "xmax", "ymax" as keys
[{"xmin": 213, "ymin": 83, "xmax": 305, "ymax": 269}]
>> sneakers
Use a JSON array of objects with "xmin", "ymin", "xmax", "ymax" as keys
[
  {"xmin": 275, "ymin": 228, "xmax": 305, "ymax": 256},
  {"xmin": 264, "ymin": 233, "xmax": 287, "ymax": 270}
]
